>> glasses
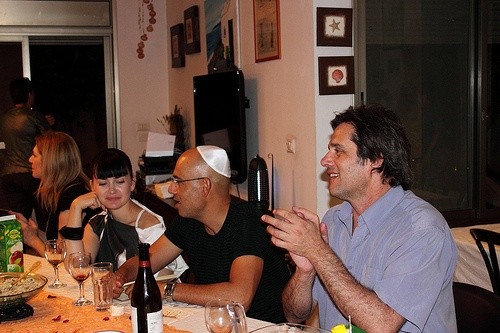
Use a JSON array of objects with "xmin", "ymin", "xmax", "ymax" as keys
[{"xmin": 171, "ymin": 173, "xmax": 209, "ymax": 186}]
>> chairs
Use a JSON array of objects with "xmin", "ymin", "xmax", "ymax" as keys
[{"xmin": 453, "ymin": 227, "xmax": 500, "ymax": 333}]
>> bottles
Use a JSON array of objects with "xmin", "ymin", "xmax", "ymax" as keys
[{"xmin": 131, "ymin": 243, "xmax": 164, "ymax": 333}]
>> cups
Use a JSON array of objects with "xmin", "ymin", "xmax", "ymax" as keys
[
  {"xmin": 205, "ymin": 298, "xmax": 235, "ymax": 333},
  {"xmin": 90, "ymin": 262, "xmax": 113, "ymax": 310}
]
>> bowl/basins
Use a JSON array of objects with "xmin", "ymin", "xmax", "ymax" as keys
[
  {"xmin": 0, "ymin": 272, "xmax": 48, "ymax": 310},
  {"xmin": 248, "ymin": 323, "xmax": 332, "ymax": 333}
]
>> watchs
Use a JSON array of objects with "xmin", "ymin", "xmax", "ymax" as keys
[{"xmin": 163, "ymin": 282, "xmax": 177, "ymax": 303}]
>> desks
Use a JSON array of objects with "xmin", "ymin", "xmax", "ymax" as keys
[{"xmin": 450, "ymin": 224, "xmax": 500, "ymax": 292}]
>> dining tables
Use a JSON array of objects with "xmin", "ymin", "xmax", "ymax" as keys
[{"xmin": 0, "ymin": 252, "xmax": 313, "ymax": 333}]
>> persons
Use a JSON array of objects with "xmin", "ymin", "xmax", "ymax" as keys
[
  {"xmin": 62, "ymin": 148, "xmax": 175, "ymax": 283},
  {"xmin": 0, "ymin": 77, "xmax": 56, "ymax": 232},
  {"xmin": 261, "ymin": 102, "xmax": 458, "ymax": 333},
  {"xmin": 7, "ymin": 130, "xmax": 90, "ymax": 261},
  {"xmin": 99, "ymin": 145, "xmax": 301, "ymax": 328}
]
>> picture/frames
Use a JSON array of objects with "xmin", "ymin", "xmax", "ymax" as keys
[
  {"xmin": 316, "ymin": 6, "xmax": 353, "ymax": 47},
  {"xmin": 169, "ymin": 23, "xmax": 185, "ymax": 69},
  {"xmin": 182, "ymin": 5, "xmax": 202, "ymax": 55},
  {"xmin": 252, "ymin": 0, "xmax": 282, "ymax": 64},
  {"xmin": 318, "ymin": 56, "xmax": 357, "ymax": 96}
]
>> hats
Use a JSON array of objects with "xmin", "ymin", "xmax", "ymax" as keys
[{"xmin": 196, "ymin": 145, "xmax": 232, "ymax": 178}]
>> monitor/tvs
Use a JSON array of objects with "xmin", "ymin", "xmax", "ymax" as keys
[{"xmin": 192, "ymin": 70, "xmax": 247, "ymax": 185}]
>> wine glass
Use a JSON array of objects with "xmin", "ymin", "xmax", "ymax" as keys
[
  {"xmin": 44, "ymin": 239, "xmax": 67, "ymax": 289},
  {"xmin": 68, "ymin": 250, "xmax": 93, "ymax": 306}
]
